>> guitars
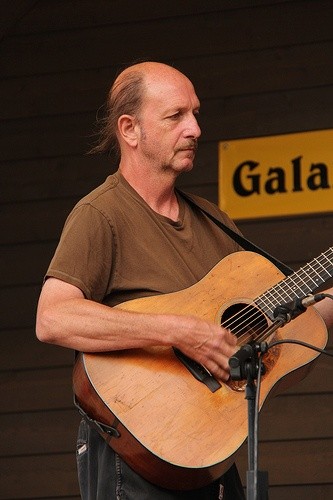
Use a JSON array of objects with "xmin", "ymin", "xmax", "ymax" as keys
[{"xmin": 72, "ymin": 246, "xmax": 333, "ymax": 486}]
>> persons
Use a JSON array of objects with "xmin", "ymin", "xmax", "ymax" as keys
[{"xmin": 35, "ymin": 61, "xmax": 333, "ymax": 500}]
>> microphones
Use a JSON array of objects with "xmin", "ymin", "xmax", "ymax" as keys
[{"xmin": 273, "ymin": 293, "xmax": 325, "ymax": 322}]
